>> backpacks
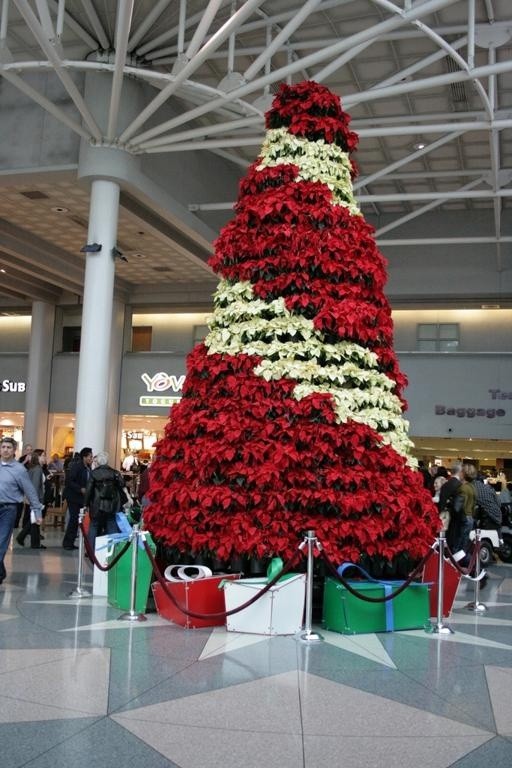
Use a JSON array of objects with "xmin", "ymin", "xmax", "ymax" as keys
[{"xmin": 90, "ymin": 476, "xmax": 122, "ymax": 522}]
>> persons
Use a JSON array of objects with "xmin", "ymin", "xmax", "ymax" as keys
[
  {"xmin": 16, "ymin": 446, "xmax": 48, "ymax": 549},
  {"xmin": 1, "ymin": 438, "xmax": 44, "ymax": 584},
  {"xmin": 48, "ymin": 447, "xmax": 149, "ymax": 557},
  {"xmin": 418, "ymin": 461, "xmax": 511, "ymax": 589}
]
